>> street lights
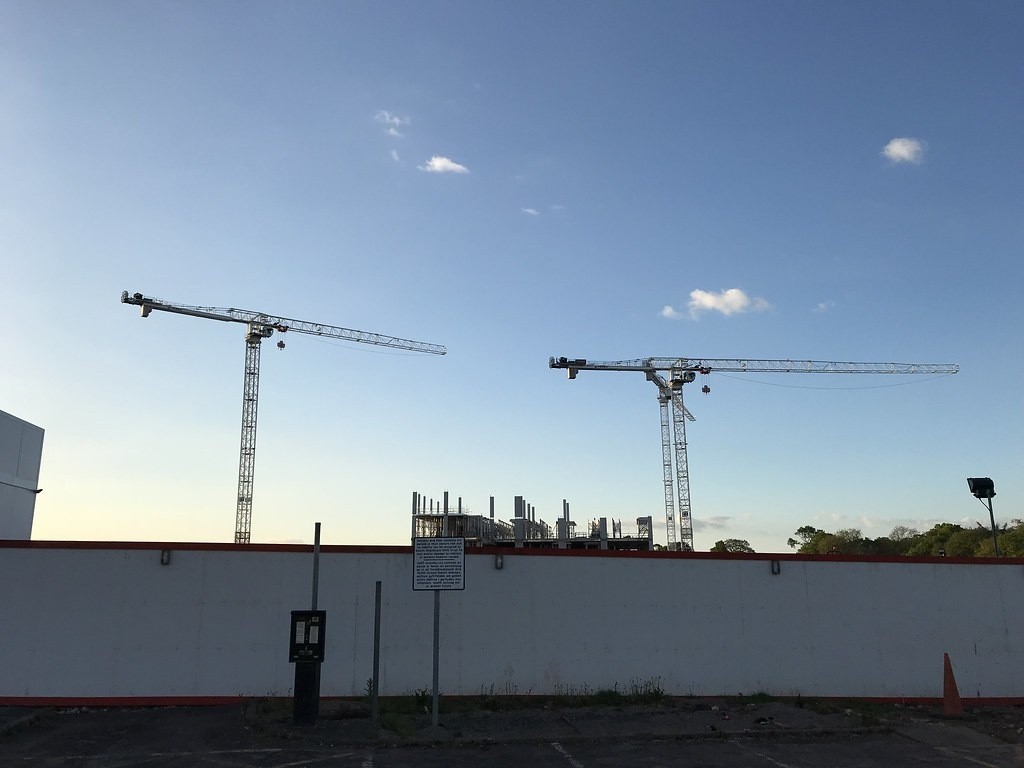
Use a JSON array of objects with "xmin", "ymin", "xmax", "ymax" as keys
[{"xmin": 966, "ymin": 477, "xmax": 1000, "ymax": 558}]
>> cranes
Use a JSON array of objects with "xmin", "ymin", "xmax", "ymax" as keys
[
  {"xmin": 121, "ymin": 290, "xmax": 447, "ymax": 553},
  {"xmin": 548, "ymin": 354, "xmax": 960, "ymax": 550}
]
